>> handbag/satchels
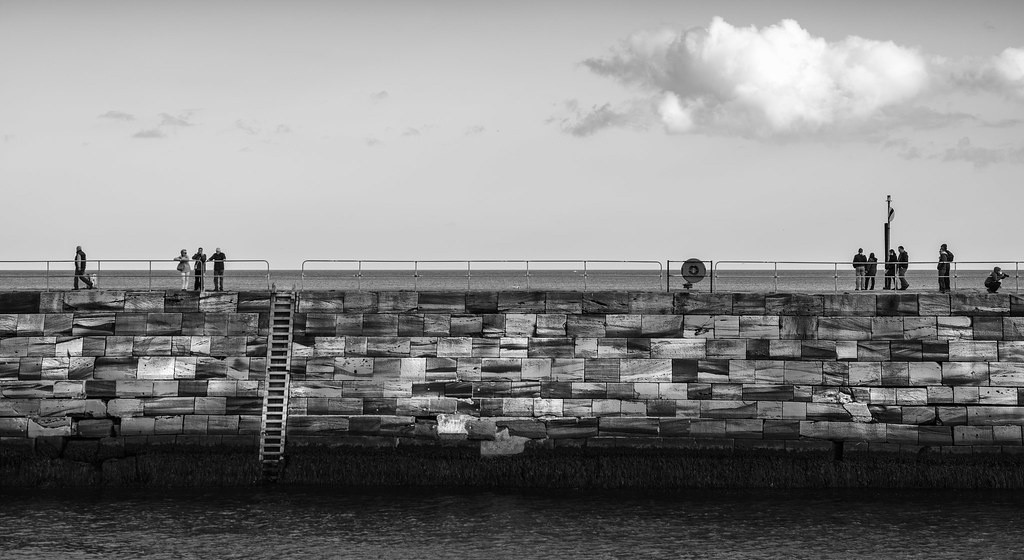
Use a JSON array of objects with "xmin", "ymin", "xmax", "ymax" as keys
[
  {"xmin": 194, "ymin": 264, "xmax": 198, "ymax": 269},
  {"xmin": 178, "ymin": 261, "xmax": 185, "ymax": 271}
]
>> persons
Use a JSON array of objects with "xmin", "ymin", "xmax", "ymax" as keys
[
  {"xmin": 174, "ymin": 249, "xmax": 191, "ymax": 289},
  {"xmin": 898, "ymin": 246, "xmax": 909, "ymax": 290},
  {"xmin": 853, "ymin": 248, "xmax": 867, "ymax": 290},
  {"xmin": 985, "ymin": 267, "xmax": 1004, "ymax": 293},
  {"xmin": 865, "ymin": 253, "xmax": 877, "ymax": 290},
  {"xmin": 192, "ymin": 248, "xmax": 206, "ymax": 291},
  {"xmin": 941, "ymin": 244, "xmax": 953, "ymax": 292},
  {"xmin": 207, "ymin": 248, "xmax": 226, "ymax": 292},
  {"xmin": 72, "ymin": 246, "xmax": 93, "ymax": 290},
  {"xmin": 937, "ymin": 247, "xmax": 948, "ymax": 292},
  {"xmin": 883, "ymin": 249, "xmax": 898, "ymax": 290}
]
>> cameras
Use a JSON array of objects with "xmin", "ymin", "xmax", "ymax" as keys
[{"xmin": 1002, "ymin": 273, "xmax": 1009, "ymax": 277}]
